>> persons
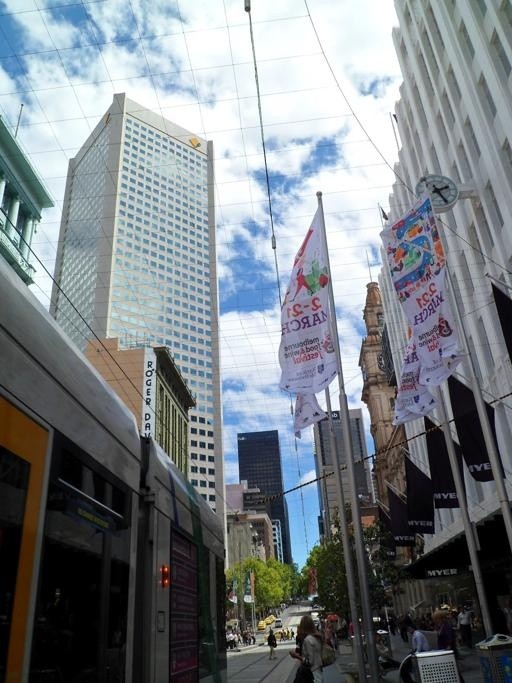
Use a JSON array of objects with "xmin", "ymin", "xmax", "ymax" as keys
[{"xmin": 226, "ymin": 598, "xmax": 512, "ymax": 683}]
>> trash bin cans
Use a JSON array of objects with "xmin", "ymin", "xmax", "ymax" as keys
[{"xmin": 475, "ymin": 634, "xmax": 512, "ymax": 683}]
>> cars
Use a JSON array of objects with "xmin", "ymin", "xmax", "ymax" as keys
[
  {"xmin": 270, "ymin": 615, "xmax": 275, "ymax": 621},
  {"xmin": 265, "ymin": 617, "xmax": 273, "ymax": 625},
  {"xmin": 258, "ymin": 621, "xmax": 266, "ymax": 629},
  {"xmin": 275, "ymin": 619, "xmax": 282, "ymax": 628},
  {"xmin": 271, "ymin": 600, "xmax": 291, "ymax": 618}
]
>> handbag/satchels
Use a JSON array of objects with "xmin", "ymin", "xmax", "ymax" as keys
[
  {"xmin": 319, "ymin": 642, "xmax": 334, "ymax": 666},
  {"xmin": 294, "ymin": 664, "xmax": 314, "ymax": 683}
]
